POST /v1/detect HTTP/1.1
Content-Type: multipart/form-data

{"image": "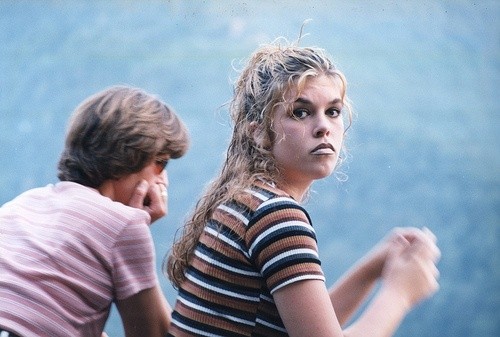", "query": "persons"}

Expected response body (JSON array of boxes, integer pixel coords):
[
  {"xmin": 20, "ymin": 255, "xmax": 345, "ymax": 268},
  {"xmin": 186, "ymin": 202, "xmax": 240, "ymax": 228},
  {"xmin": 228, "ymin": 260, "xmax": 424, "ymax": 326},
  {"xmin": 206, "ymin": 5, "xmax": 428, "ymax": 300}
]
[
  {"xmin": 167, "ymin": 19, "xmax": 442, "ymax": 336},
  {"xmin": 0, "ymin": 85, "xmax": 191, "ymax": 336}
]
